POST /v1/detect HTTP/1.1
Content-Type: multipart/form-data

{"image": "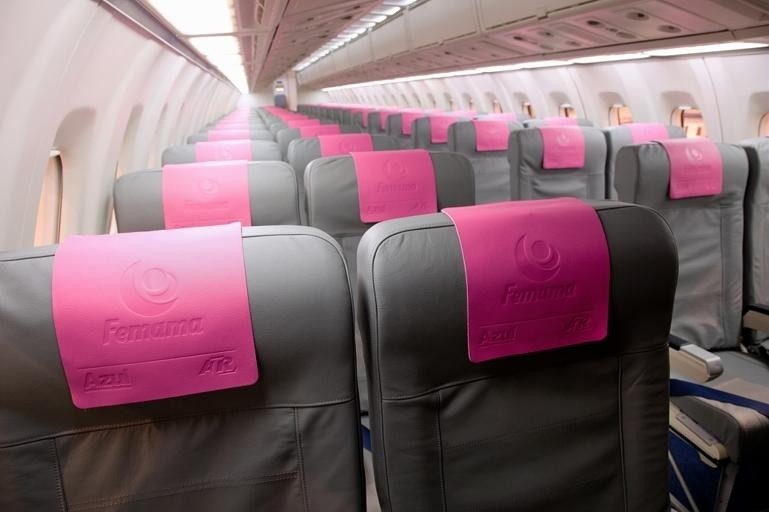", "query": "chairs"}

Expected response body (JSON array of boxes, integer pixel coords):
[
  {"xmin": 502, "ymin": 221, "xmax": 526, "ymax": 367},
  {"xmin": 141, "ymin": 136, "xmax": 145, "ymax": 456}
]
[
  {"xmin": 256, "ymin": 105, "xmax": 319, "ymax": 129},
  {"xmin": 385, "ymin": 111, "xmax": 430, "ymax": 149},
  {"xmin": 474, "ymin": 113, "xmax": 532, "ymax": 127},
  {"xmin": 196, "ymin": 107, "xmax": 265, "ymax": 125},
  {"xmin": 354, "ymin": 110, "xmax": 368, "ymax": 133},
  {"xmin": 161, "ymin": 139, "xmax": 282, "ymax": 169},
  {"xmin": 275, "ymin": 123, "xmax": 361, "ymax": 162},
  {"xmin": 367, "ymin": 109, "xmax": 401, "ymax": 133},
  {"xmin": 613, "ymin": 135, "xmax": 769, "ymax": 512},
  {"xmin": 447, "ymin": 120, "xmax": 526, "ymax": 205},
  {"xmin": 429, "ymin": 109, "xmax": 448, "ymax": 114},
  {"xmin": 522, "ymin": 116, "xmax": 593, "ymax": 128},
  {"xmin": 0, "ymin": 222, "xmax": 367, "ymax": 512},
  {"xmin": 286, "ymin": 134, "xmax": 400, "ymax": 228},
  {"xmin": 187, "ymin": 130, "xmax": 277, "ymax": 144},
  {"xmin": 601, "ymin": 122, "xmax": 686, "ymax": 201},
  {"xmin": 343, "ymin": 109, "xmax": 357, "ymax": 125},
  {"xmin": 736, "ymin": 136, "xmax": 769, "ymax": 363},
  {"xmin": 302, "ymin": 146, "xmax": 476, "ymax": 512},
  {"xmin": 297, "ymin": 104, "xmax": 344, "ymax": 125},
  {"xmin": 112, "ymin": 158, "xmax": 301, "ymax": 233},
  {"xmin": 269, "ymin": 118, "xmax": 337, "ymax": 143},
  {"xmin": 356, "ymin": 197, "xmax": 679, "ymax": 511},
  {"xmin": 199, "ymin": 125, "xmax": 267, "ymax": 135},
  {"xmin": 411, "ymin": 115, "xmax": 471, "ymax": 152},
  {"xmin": 507, "ymin": 126, "xmax": 607, "ymax": 202}
]
